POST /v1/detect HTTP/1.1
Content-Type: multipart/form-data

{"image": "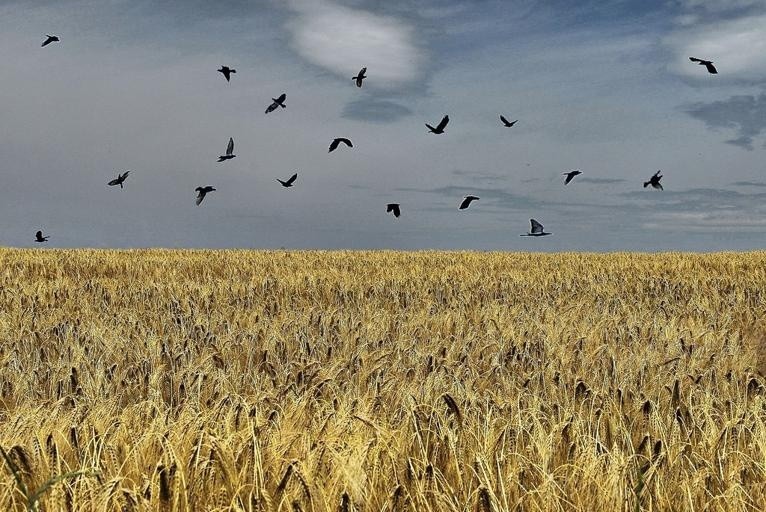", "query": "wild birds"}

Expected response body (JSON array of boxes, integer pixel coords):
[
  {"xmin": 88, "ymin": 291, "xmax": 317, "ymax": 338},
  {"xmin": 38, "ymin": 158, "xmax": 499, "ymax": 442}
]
[
  {"xmin": 40, "ymin": 33, "xmax": 58, "ymax": 50},
  {"xmin": 33, "ymin": 229, "xmax": 49, "ymax": 246},
  {"xmin": 192, "ymin": 66, "xmax": 369, "ymax": 207},
  {"xmin": 459, "ymin": 193, "xmax": 480, "ymax": 212},
  {"xmin": 384, "ymin": 202, "xmax": 403, "ymax": 220},
  {"xmin": 423, "ymin": 113, "xmax": 452, "ymax": 138},
  {"xmin": 642, "ymin": 168, "xmax": 665, "ymax": 192},
  {"xmin": 521, "ymin": 218, "xmax": 556, "ymax": 240},
  {"xmin": 561, "ymin": 168, "xmax": 580, "ymax": 187},
  {"xmin": 497, "ymin": 114, "xmax": 520, "ymax": 131},
  {"xmin": 690, "ymin": 55, "xmax": 718, "ymax": 76},
  {"xmin": 107, "ymin": 171, "xmax": 129, "ymax": 191}
]
